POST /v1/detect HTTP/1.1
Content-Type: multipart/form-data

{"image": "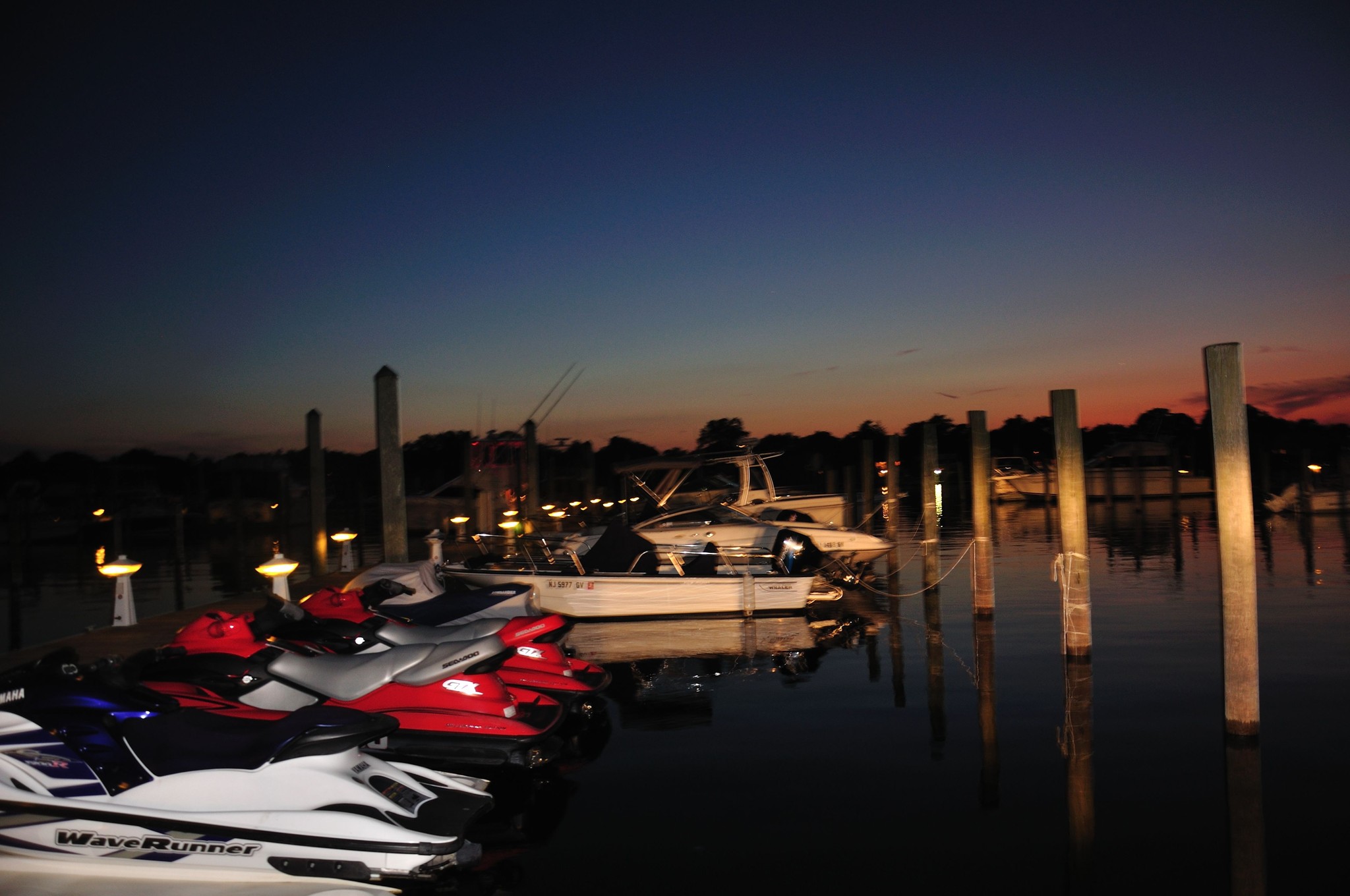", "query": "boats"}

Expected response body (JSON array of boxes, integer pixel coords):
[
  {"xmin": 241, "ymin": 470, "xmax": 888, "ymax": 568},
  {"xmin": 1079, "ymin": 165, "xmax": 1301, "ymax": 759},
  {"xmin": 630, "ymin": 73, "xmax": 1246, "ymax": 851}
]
[
  {"xmin": 1265, "ymin": 480, "xmax": 1350, "ymax": 519},
  {"xmin": 984, "ymin": 453, "xmax": 1215, "ymax": 501},
  {"xmin": 0, "ymin": 446, "xmax": 915, "ymax": 896}
]
